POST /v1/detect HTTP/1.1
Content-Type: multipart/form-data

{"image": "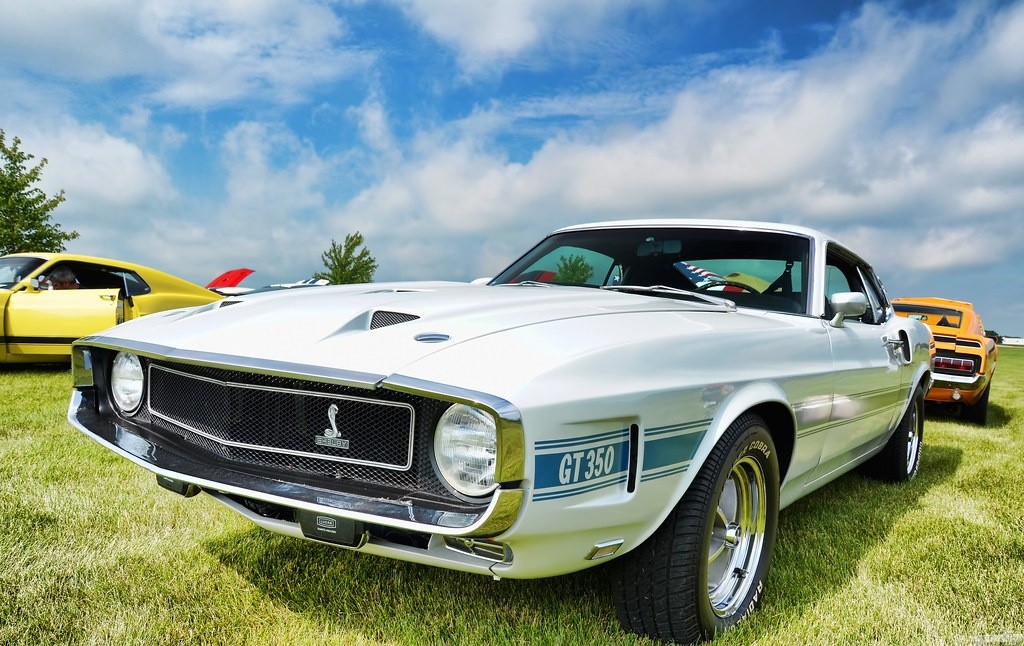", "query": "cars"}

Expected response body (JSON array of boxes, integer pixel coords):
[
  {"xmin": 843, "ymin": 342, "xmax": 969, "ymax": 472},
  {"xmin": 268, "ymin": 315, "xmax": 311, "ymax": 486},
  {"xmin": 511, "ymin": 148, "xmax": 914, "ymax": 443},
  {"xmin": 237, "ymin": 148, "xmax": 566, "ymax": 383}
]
[
  {"xmin": 889, "ymin": 296, "xmax": 999, "ymax": 427},
  {"xmin": 0, "ymin": 252, "xmax": 229, "ymax": 374},
  {"xmin": 63, "ymin": 219, "xmax": 937, "ymax": 645}
]
